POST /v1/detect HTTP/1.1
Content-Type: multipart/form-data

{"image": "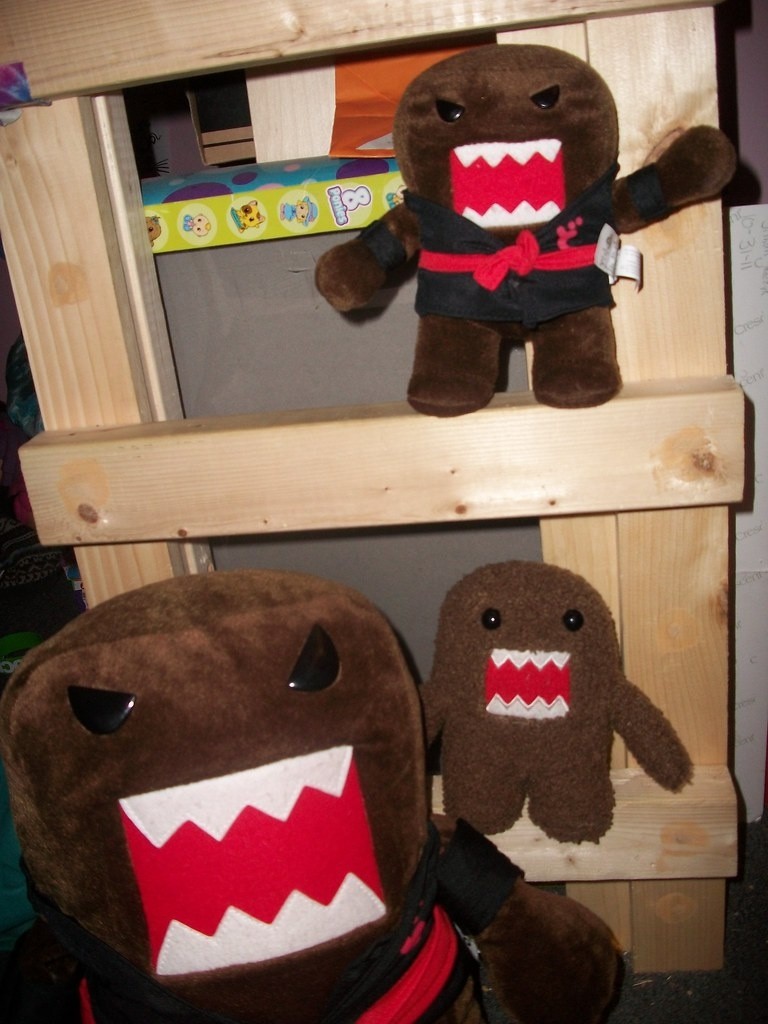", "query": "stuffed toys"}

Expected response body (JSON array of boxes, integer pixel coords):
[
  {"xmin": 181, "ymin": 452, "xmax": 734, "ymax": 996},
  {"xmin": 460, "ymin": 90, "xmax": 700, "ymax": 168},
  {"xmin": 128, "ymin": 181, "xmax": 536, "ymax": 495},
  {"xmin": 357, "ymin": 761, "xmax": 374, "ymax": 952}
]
[
  {"xmin": 313, "ymin": 44, "xmax": 738, "ymax": 419},
  {"xmin": 418, "ymin": 561, "xmax": 692, "ymax": 842},
  {"xmin": 0, "ymin": 568, "xmax": 626, "ymax": 1023}
]
